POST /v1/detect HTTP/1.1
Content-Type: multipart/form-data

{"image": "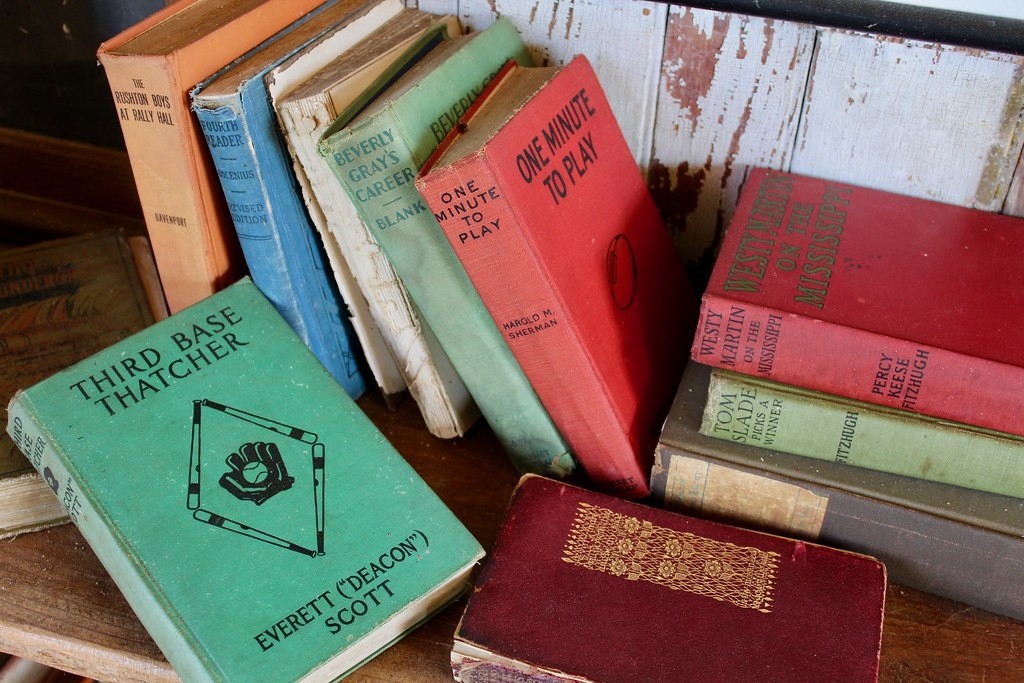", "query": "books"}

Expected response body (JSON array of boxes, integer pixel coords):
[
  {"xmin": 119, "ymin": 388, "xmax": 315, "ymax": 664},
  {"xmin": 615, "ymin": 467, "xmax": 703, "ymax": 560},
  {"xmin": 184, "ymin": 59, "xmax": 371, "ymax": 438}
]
[
  {"xmin": 97, "ymin": 0, "xmax": 692, "ymax": 500},
  {"xmin": 0, "ymin": 228, "xmax": 168, "ymax": 540},
  {"xmin": 451, "ymin": 472, "xmax": 888, "ymax": 683},
  {"xmin": 649, "ymin": 168, "xmax": 1024, "ymax": 621},
  {"xmin": 4, "ymin": 276, "xmax": 487, "ymax": 683}
]
